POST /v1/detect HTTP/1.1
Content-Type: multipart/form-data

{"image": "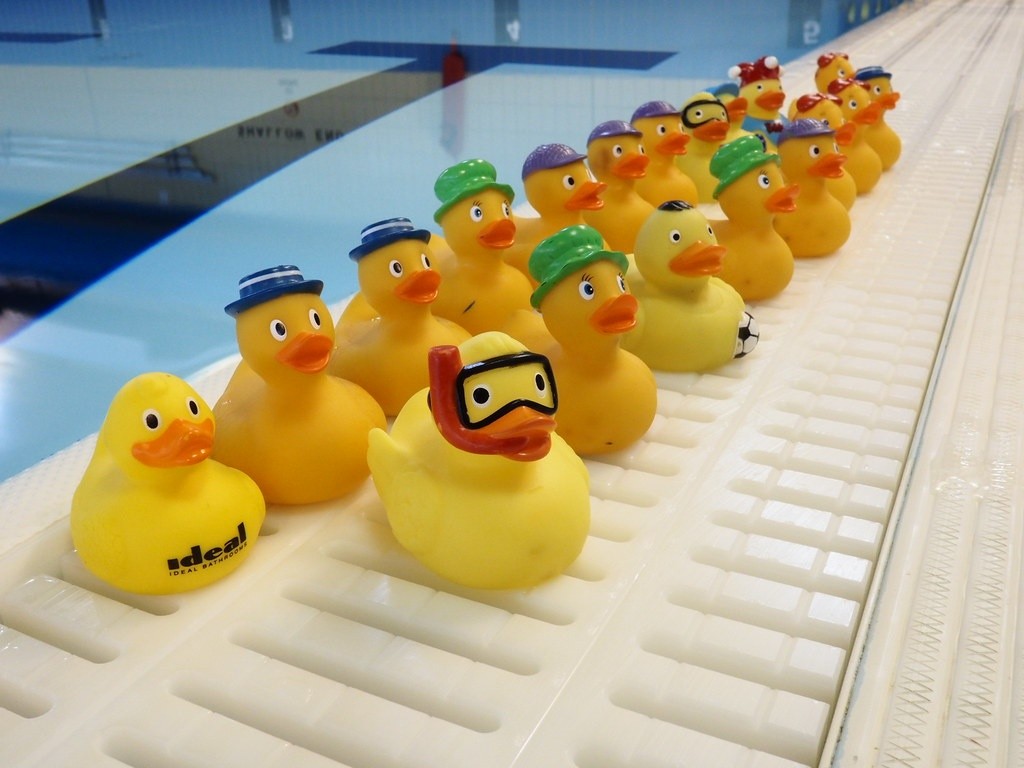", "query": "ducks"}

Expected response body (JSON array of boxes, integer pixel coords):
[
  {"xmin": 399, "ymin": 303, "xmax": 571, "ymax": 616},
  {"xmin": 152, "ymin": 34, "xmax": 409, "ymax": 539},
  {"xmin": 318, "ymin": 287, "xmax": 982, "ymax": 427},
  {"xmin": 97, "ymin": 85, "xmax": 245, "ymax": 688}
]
[{"xmin": 70, "ymin": 53, "xmax": 900, "ymax": 596}]
